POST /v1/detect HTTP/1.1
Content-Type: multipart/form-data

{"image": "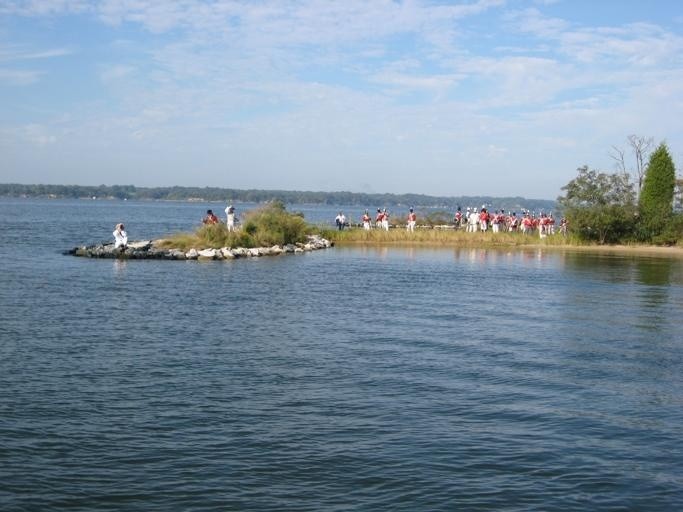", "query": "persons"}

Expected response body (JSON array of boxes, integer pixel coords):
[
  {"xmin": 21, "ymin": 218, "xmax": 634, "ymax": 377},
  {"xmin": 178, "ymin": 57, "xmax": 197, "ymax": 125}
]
[
  {"xmin": 406, "ymin": 208, "xmax": 416, "ymax": 232},
  {"xmin": 381, "ymin": 209, "xmax": 388, "ymax": 231},
  {"xmin": 362, "ymin": 209, "xmax": 371, "ymax": 230},
  {"xmin": 111, "ymin": 223, "xmax": 127, "ymax": 247},
  {"xmin": 199, "ymin": 209, "xmax": 216, "ymax": 227},
  {"xmin": 334, "ymin": 211, "xmax": 344, "ymax": 231},
  {"xmin": 467, "ymin": 247, "xmax": 543, "ymax": 267},
  {"xmin": 373, "ymin": 208, "xmax": 384, "ymax": 229},
  {"xmin": 360, "ymin": 244, "xmax": 416, "ymax": 259},
  {"xmin": 453, "ymin": 206, "xmax": 567, "ymax": 239},
  {"xmin": 224, "ymin": 205, "xmax": 238, "ymax": 234},
  {"xmin": 112, "ymin": 259, "xmax": 126, "ymax": 278}
]
